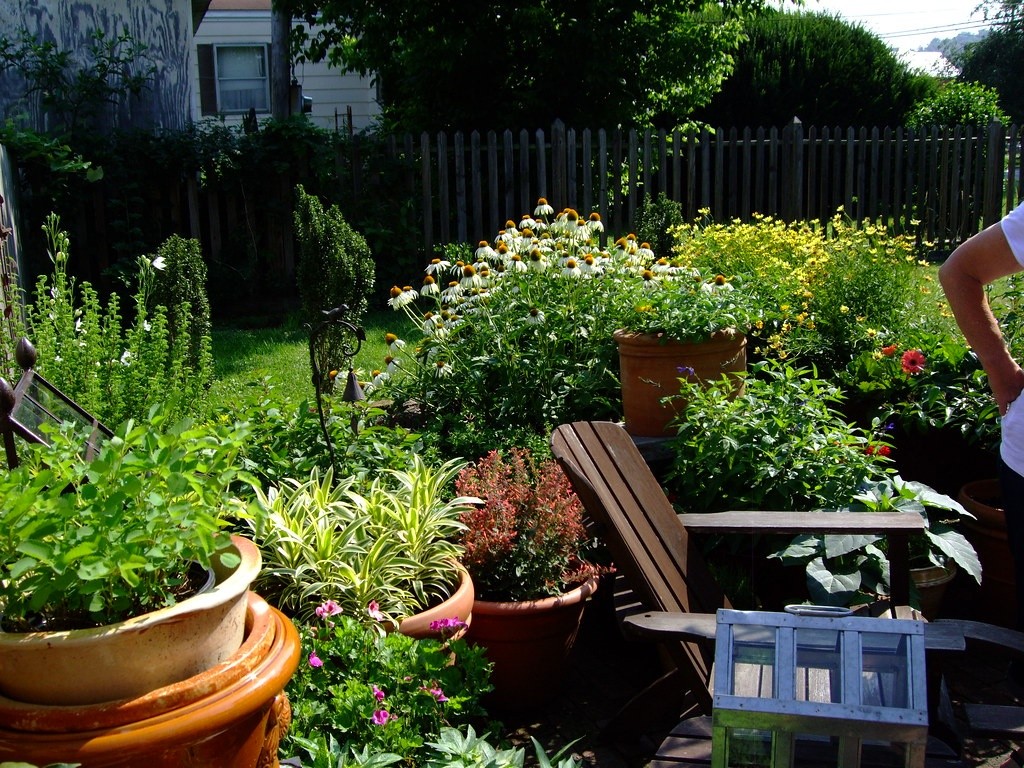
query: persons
[939,195,1024,705]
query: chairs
[549,421,966,731]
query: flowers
[608,276,764,346]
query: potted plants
[0,401,272,708]
[876,352,1023,616]
[450,446,618,701]
[848,476,984,623]
[230,452,484,671]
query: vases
[612,323,750,439]
